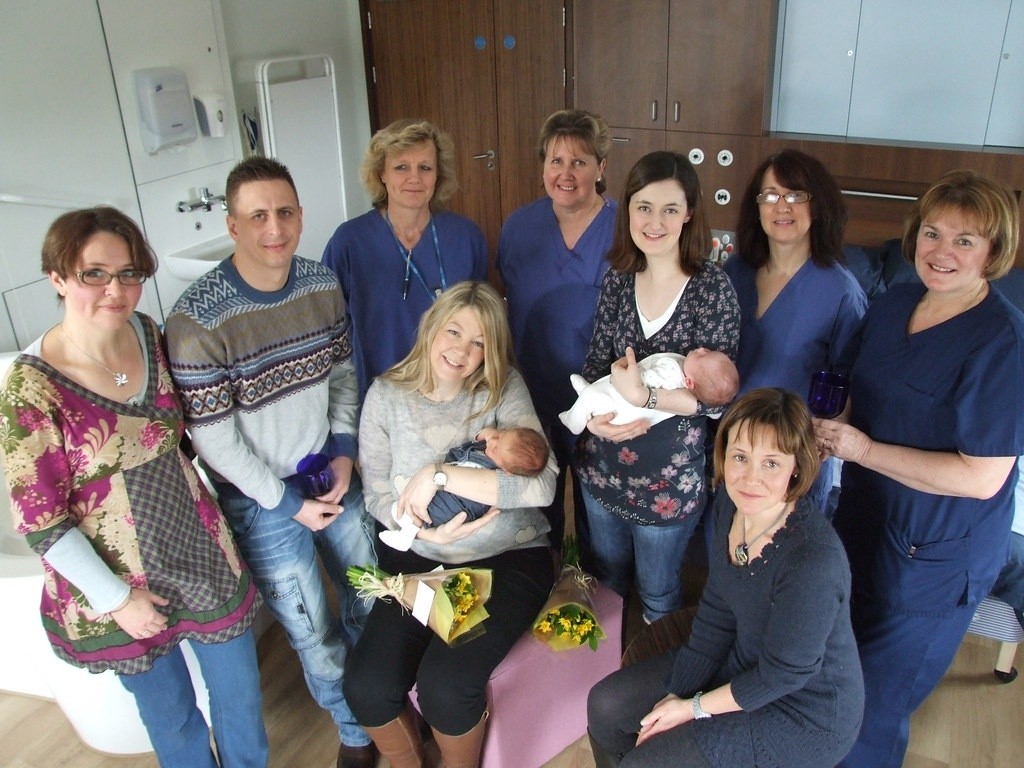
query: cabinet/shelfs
[501,1,566,230]
[759,136,1023,271]
[775,0,1010,154]
[984,0,1024,154]
[602,126,666,206]
[369,0,500,281]
[575,1,777,137]
[666,130,761,230]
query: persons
[558,346,740,435]
[497,110,619,569]
[570,152,741,638]
[704,149,867,576]
[318,119,488,413]
[810,171,1024,768]
[378,426,550,552]
[165,157,428,768]
[586,388,865,768]
[342,284,559,768]
[0,208,268,768]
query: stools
[402,564,624,767]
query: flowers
[533,533,606,650]
[347,561,489,644]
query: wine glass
[297,453,344,518]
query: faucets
[176,186,227,213]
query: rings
[822,440,826,447]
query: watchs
[692,691,712,720]
[433,463,447,491]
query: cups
[809,370,850,418]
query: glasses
[73,269,147,286]
[756,192,812,205]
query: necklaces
[736,499,791,565]
[60,324,127,385]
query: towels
[241,112,261,160]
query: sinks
[165,230,237,281]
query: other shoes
[337,742,377,768]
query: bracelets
[112,588,132,613]
[642,387,657,409]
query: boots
[428,701,489,768]
[361,695,432,768]
